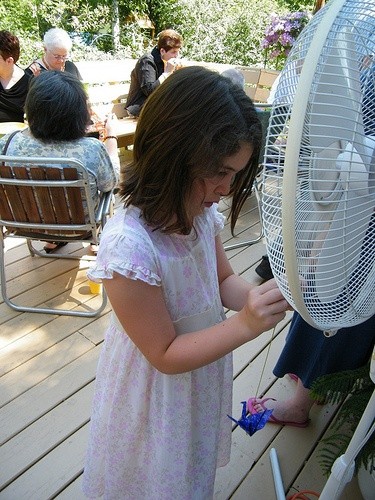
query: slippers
[248,396,311,428]
[289,372,327,406]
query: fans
[254,0,375,500]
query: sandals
[43,241,68,253]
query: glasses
[51,55,70,61]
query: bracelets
[103,136,119,141]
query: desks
[102,115,140,148]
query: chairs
[0,155,113,319]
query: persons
[0,29,34,124]
[247,68,375,429]
[76,64,292,500]
[25,28,92,125]
[0,70,121,253]
[124,29,182,116]
